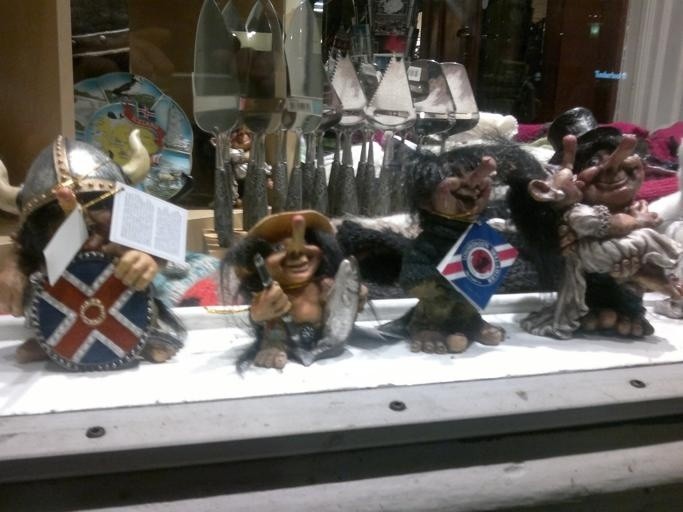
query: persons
[0,127,190,374]
[338,144,505,356]
[497,134,682,321]
[520,106,662,339]
[207,120,277,206]
[217,204,370,371]
